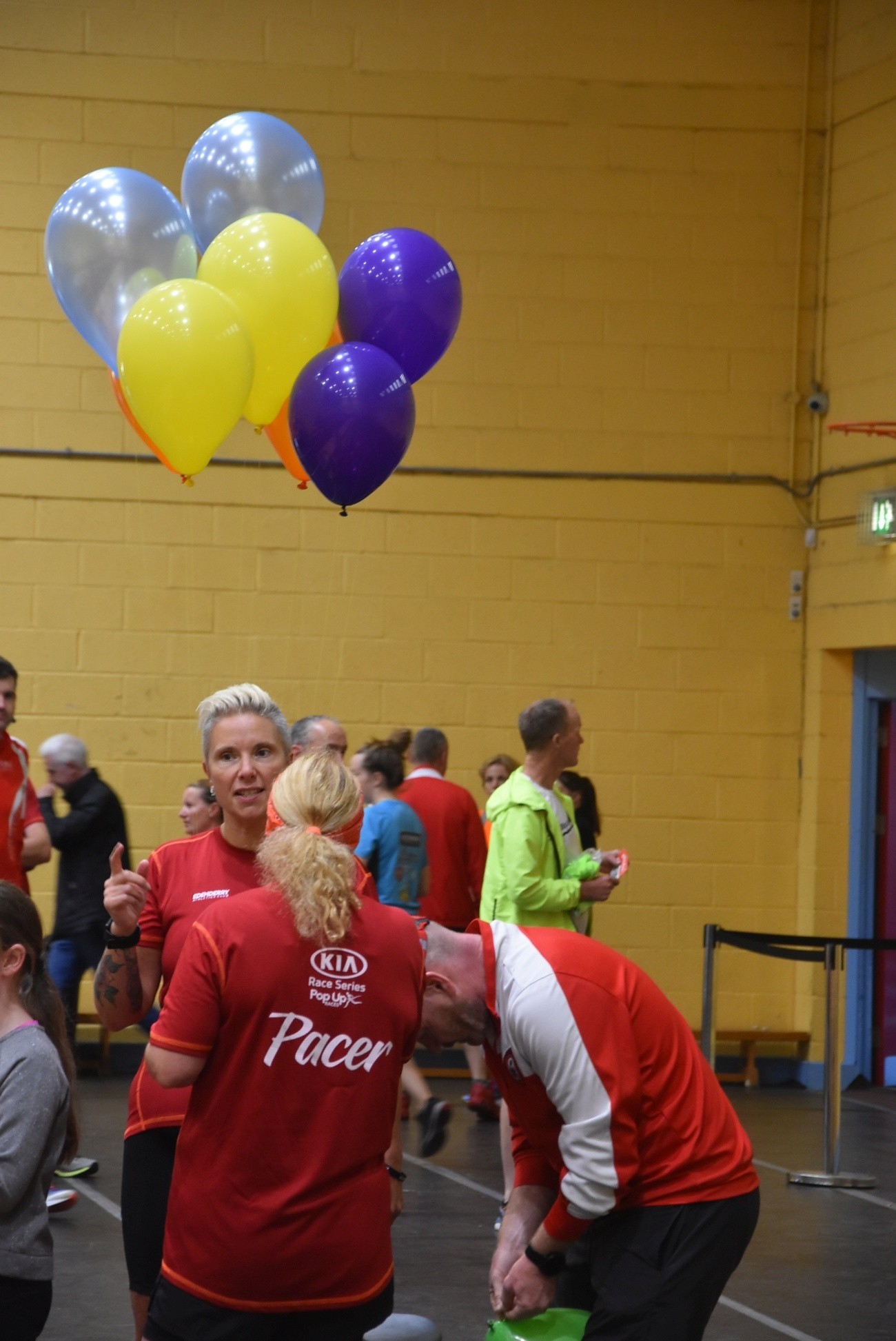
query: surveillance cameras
[807,392,827,414]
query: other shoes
[493,1201,508,1229]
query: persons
[0,878,80,1341]
[143,747,426,1341]
[94,682,379,1341]
[416,916,762,1341]
[0,655,602,1223]
[480,695,623,938]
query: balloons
[42,110,463,519]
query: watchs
[523,1240,567,1279]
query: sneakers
[54,1157,98,1177]
[415,1096,452,1157]
[400,1095,410,1121]
[46,1185,77,1212]
[467,1082,501,1120]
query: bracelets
[104,918,143,951]
[383,1162,408,1182]
